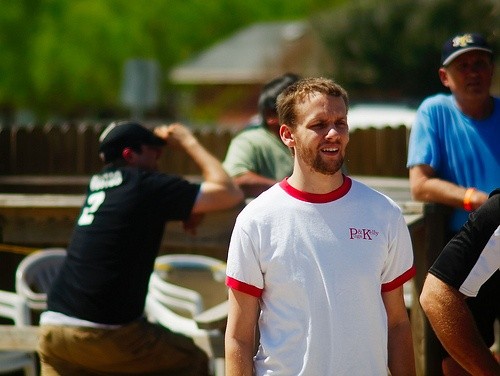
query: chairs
[0,246,229,376]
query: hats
[441,32,493,70]
[100,120,168,165]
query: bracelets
[463,188,475,211]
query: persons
[223,72,299,200]
[35,118,246,376]
[406,27,500,376]
[419,188,500,376]
[223,77,416,375]
